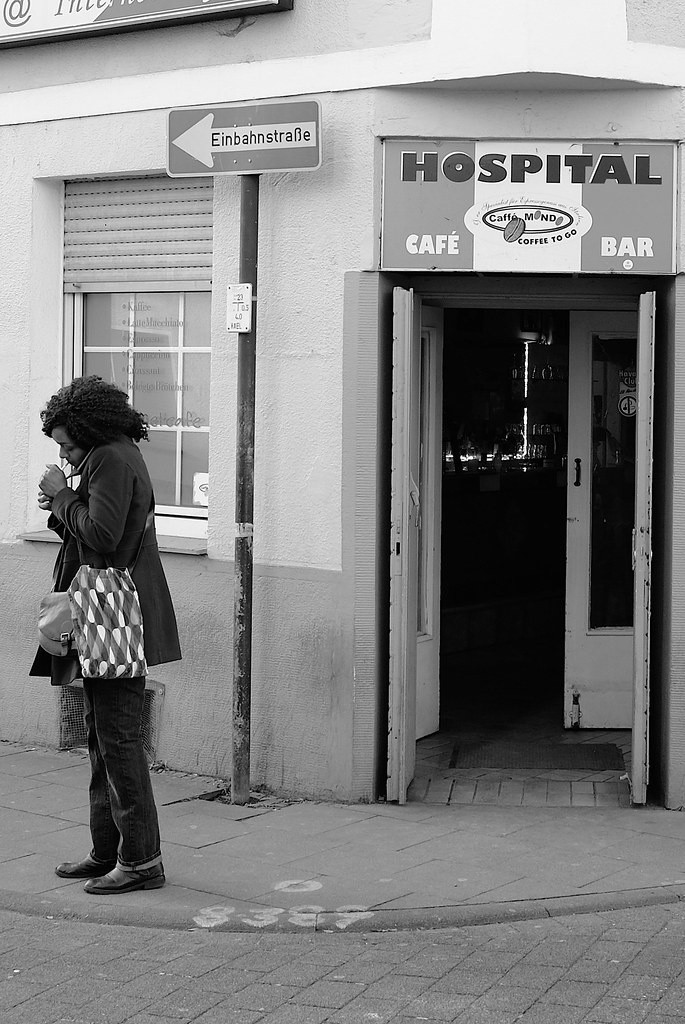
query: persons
[28,377,181,896]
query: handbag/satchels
[38,592,75,656]
[74,511,150,678]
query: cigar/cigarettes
[62,461,69,470]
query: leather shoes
[55,853,117,878]
[83,861,165,895]
